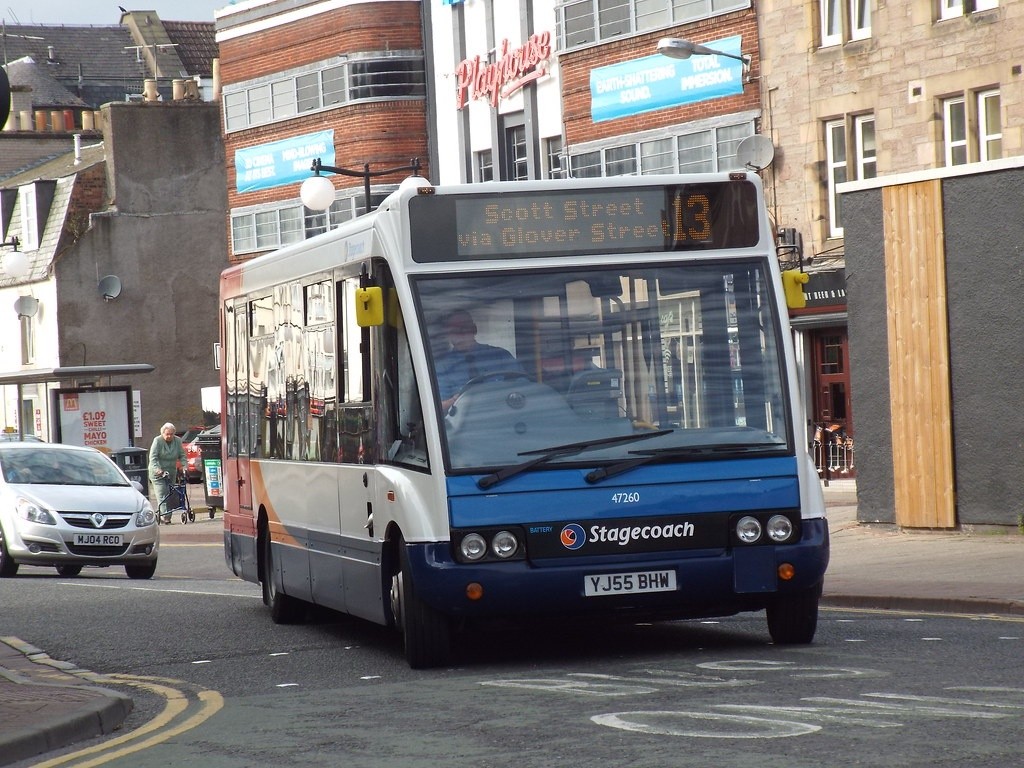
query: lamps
[656,37,752,73]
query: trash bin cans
[105,445,150,503]
[194,426,223,520]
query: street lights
[299,159,434,216]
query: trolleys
[155,471,197,524]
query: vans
[176,425,211,484]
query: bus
[216,165,830,669]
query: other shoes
[160,516,171,524]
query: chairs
[568,366,623,416]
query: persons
[432,310,526,412]
[149,422,187,524]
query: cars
[0,441,161,580]
[0,432,45,442]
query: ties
[465,357,482,384]
